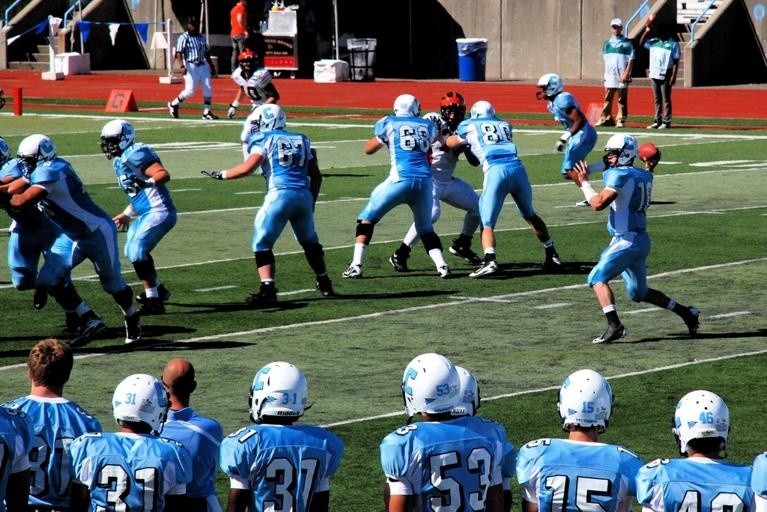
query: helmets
[400,352,461,418]
[250,103,287,135]
[671,387,731,459]
[14,134,57,179]
[111,373,173,437]
[440,90,466,117]
[97,117,136,160]
[450,365,482,417]
[0,136,11,167]
[393,93,420,118]
[556,369,615,435]
[470,100,496,119]
[536,72,564,100]
[602,133,638,170]
[248,360,310,425]
[237,48,258,72]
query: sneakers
[591,324,627,345]
[437,265,454,279]
[135,298,167,317]
[387,248,410,272]
[683,305,701,338]
[542,253,564,269]
[468,253,499,279]
[33,288,48,310]
[245,281,278,307]
[614,120,624,128]
[313,271,337,298]
[657,122,670,130]
[167,101,181,119]
[646,122,658,129]
[66,310,107,349]
[594,118,610,127]
[135,284,171,302]
[576,199,590,206]
[201,110,219,120]
[448,238,483,266]
[123,311,143,345]
[341,263,364,279]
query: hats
[610,18,622,27]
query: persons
[536,73,610,179]
[388,92,480,272]
[226,52,280,174]
[70,373,187,512]
[0,403,32,512]
[341,93,451,279]
[219,362,344,512]
[231,0,249,73]
[245,25,268,67]
[515,369,645,512]
[640,20,681,131]
[595,19,636,128]
[0,134,141,351]
[451,366,516,512]
[150,357,222,512]
[201,104,334,305]
[635,390,755,512]
[378,353,495,512]
[447,101,563,279]
[168,18,219,122]
[574,134,700,344]
[0,136,80,331]
[101,119,177,317]
[5,339,102,512]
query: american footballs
[635,141,662,163]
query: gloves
[554,130,571,152]
[130,174,156,189]
[226,104,238,119]
[200,169,228,180]
